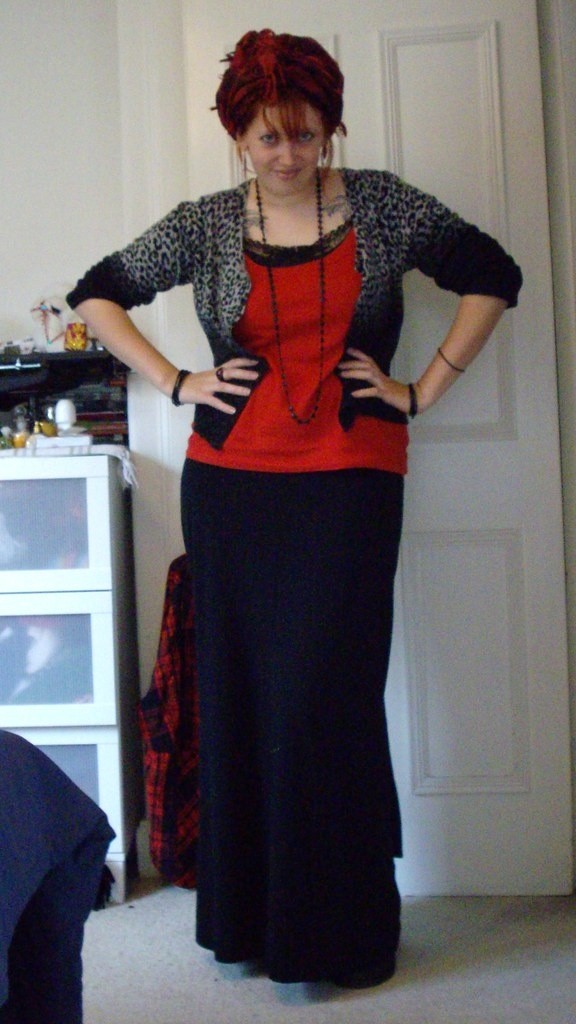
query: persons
[65,30,525,1002]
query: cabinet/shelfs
[0,452,143,907]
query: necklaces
[254,168,326,425]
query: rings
[216,367,224,382]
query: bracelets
[409,384,417,420]
[171,370,192,407]
[437,347,464,373]
